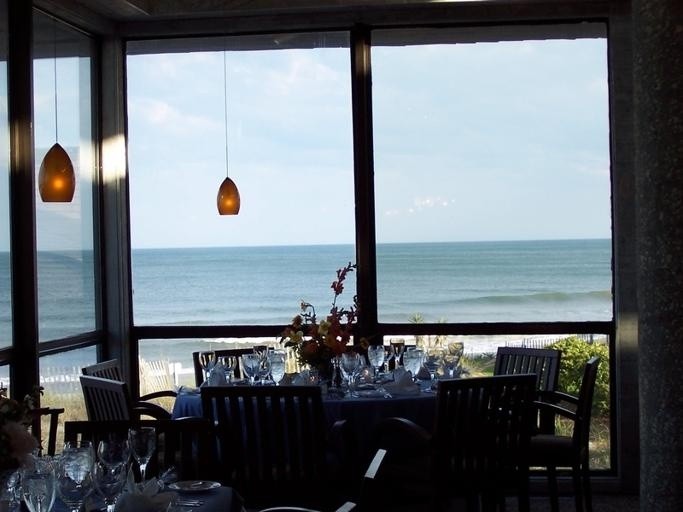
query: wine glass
[170,480,222,492]
[403,342,464,392]
[368,346,385,384]
[199,353,216,386]
[20,440,131,512]
[242,353,286,385]
[220,358,237,387]
[381,345,393,375]
[339,353,366,399]
[128,427,156,487]
[392,340,405,371]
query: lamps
[217,0,240,216]
[38,1,75,202]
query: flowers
[277,261,380,384]
